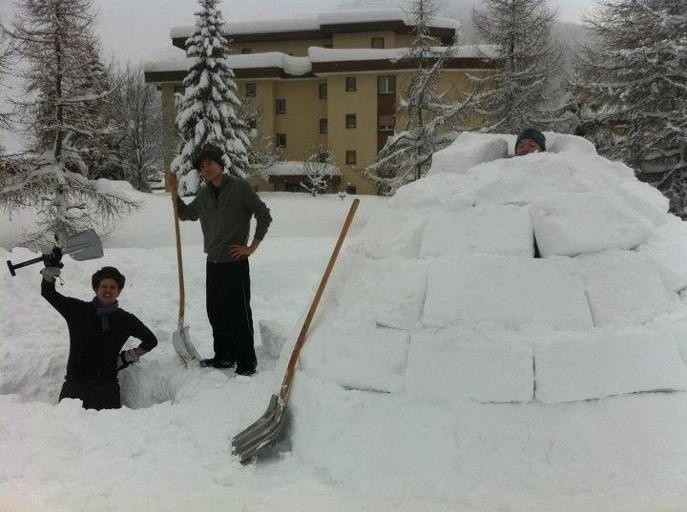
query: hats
[192,144,224,168]
[93,267,125,289]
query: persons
[514,128,547,156]
[40,246,158,413]
[165,150,273,377]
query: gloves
[116,348,139,370]
[40,248,64,282]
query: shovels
[231,198,360,464]
[170,172,202,368]
[7,228,104,277]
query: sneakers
[235,359,257,375]
[201,353,235,368]
